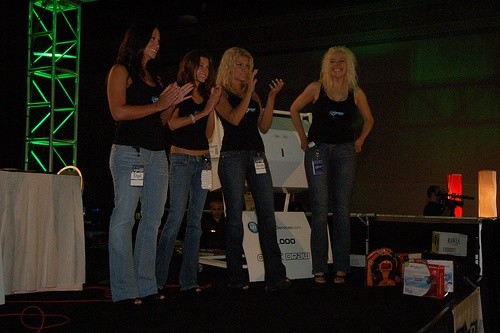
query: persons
[423,185,447,216]
[156,50,220,295]
[205,201,226,248]
[106,23,194,306]
[290,47,374,283]
[214,48,289,292]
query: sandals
[333,274,347,283]
[314,271,327,283]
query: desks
[0,169,85,305]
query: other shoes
[113,299,145,308]
[264,278,291,293]
[224,278,250,292]
[158,287,168,299]
[144,294,169,304]
[179,285,204,297]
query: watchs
[189,114,196,124]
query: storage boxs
[429,230,468,258]
[402,262,446,299]
